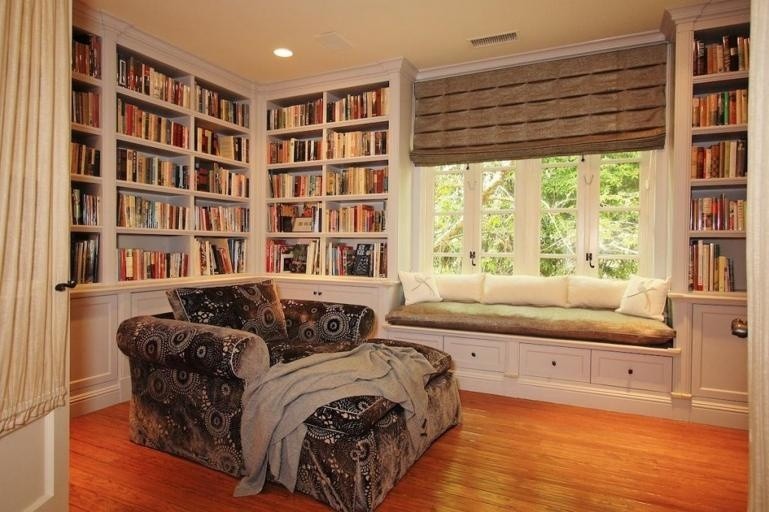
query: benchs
[385,301,680,421]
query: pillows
[399,270,670,322]
[165,278,295,364]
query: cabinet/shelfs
[670,3,750,429]
[71,20,110,389]
[108,32,259,376]
[261,78,396,335]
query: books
[266,87,389,279]
[70,38,101,285]
[195,84,251,276]
[116,55,191,282]
[687,34,749,293]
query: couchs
[108,297,462,511]
